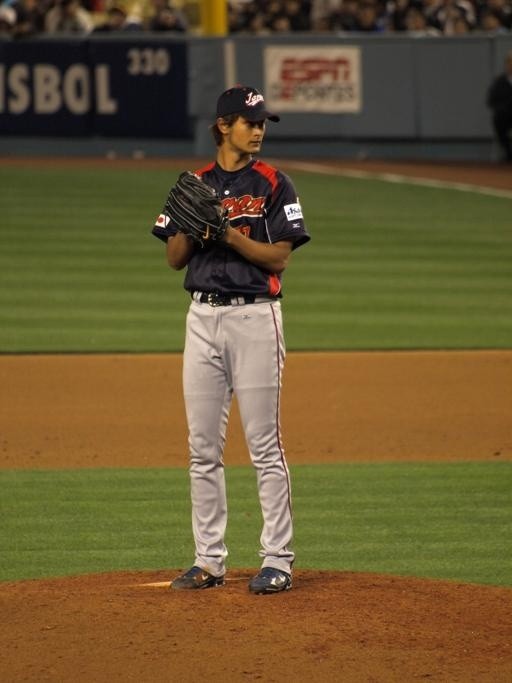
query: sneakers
[168,562,228,592]
[248,562,293,596]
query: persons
[485,54,512,163]
[151,85,310,591]
[1,1,511,41]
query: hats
[214,85,280,123]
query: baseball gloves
[162,171,229,248]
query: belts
[191,289,279,306]
